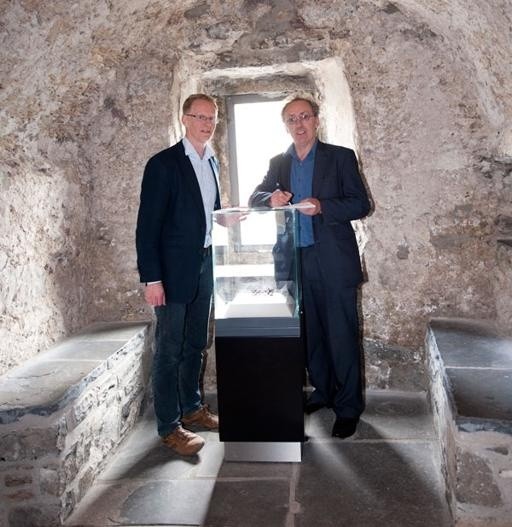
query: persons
[247,95,376,439]
[133,93,252,456]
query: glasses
[185,113,219,124]
[287,112,316,124]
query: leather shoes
[332,416,359,439]
[305,397,323,414]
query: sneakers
[181,404,219,432]
[160,426,204,456]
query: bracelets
[146,280,162,286]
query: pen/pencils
[276,182,291,205]
